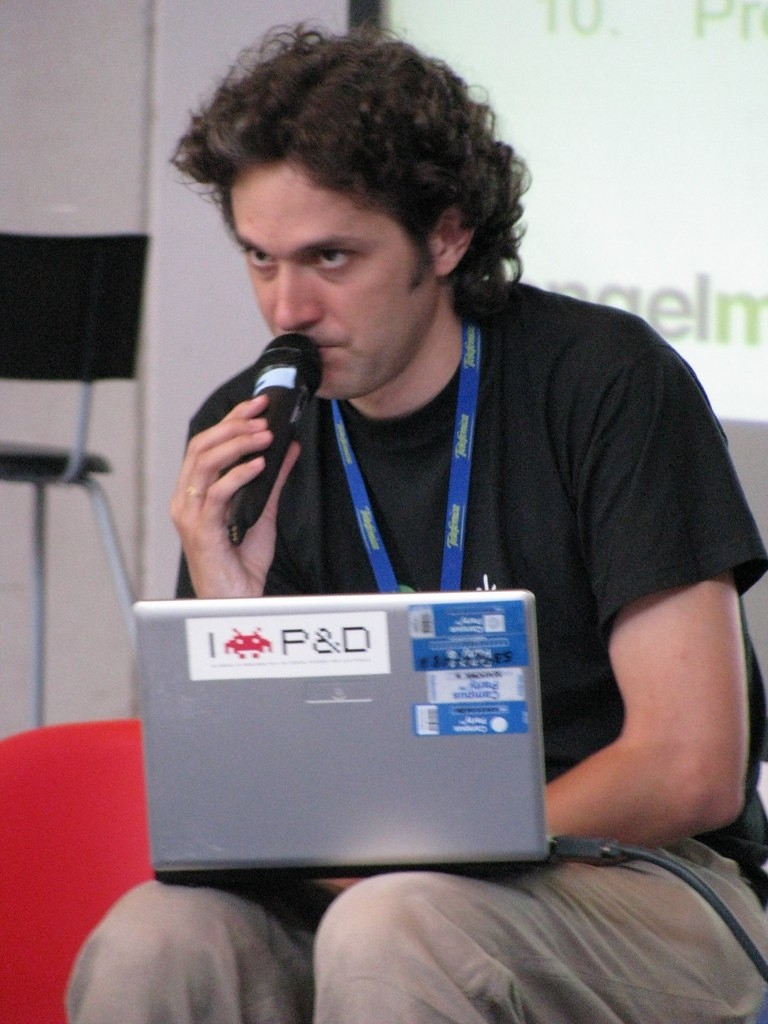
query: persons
[65,23,768,1024]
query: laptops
[130,590,559,885]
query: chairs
[1,233,151,728]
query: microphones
[228,332,324,545]
